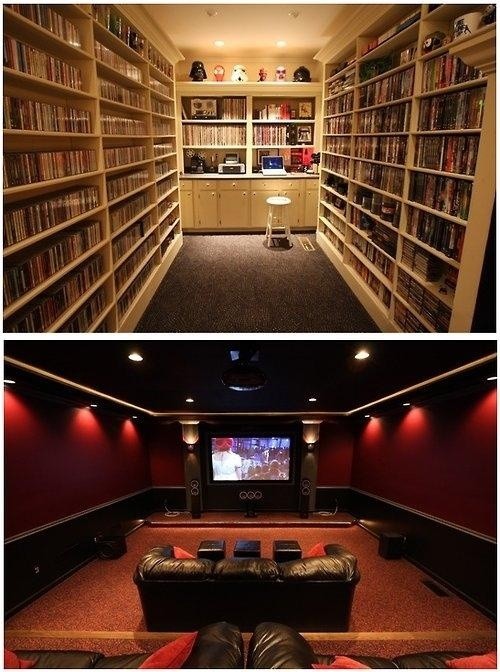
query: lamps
[354,347,372,363]
[127,348,143,363]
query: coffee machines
[190,154,205,174]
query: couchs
[133,535,362,635]
[250,618,496,669]
[5,613,245,668]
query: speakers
[190,478,202,519]
[239,491,264,501]
[300,478,311,519]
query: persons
[211,438,242,480]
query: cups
[99,6,144,57]
[453,12,483,40]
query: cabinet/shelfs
[173,73,323,173]
[3,3,190,333]
[178,172,322,238]
[307,3,496,330]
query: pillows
[443,646,496,669]
[4,645,41,669]
[172,546,195,560]
[310,650,379,669]
[301,543,328,559]
[138,625,202,668]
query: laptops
[261,155,288,176]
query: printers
[218,161,246,174]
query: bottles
[292,66,310,82]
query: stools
[261,194,298,250]
[271,538,305,563]
[234,537,265,558]
[377,529,407,562]
[196,538,226,563]
